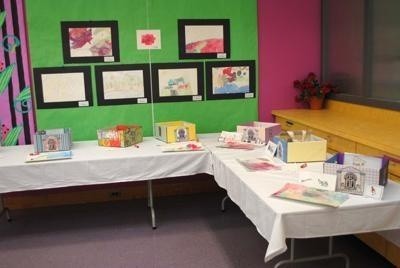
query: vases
[309,96,324,108]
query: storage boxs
[33,128,72,153]
[154,121,195,144]
[322,152,389,187]
[97,125,142,148]
[236,122,282,145]
[274,135,327,163]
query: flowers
[293,72,338,101]
[329,72,351,92]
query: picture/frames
[94,63,152,106]
[136,30,161,50]
[205,60,256,100]
[33,66,93,110]
[177,18,230,59]
[60,20,120,64]
[151,62,204,103]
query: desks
[0,137,213,230]
[271,100,399,183]
[197,132,400,268]
